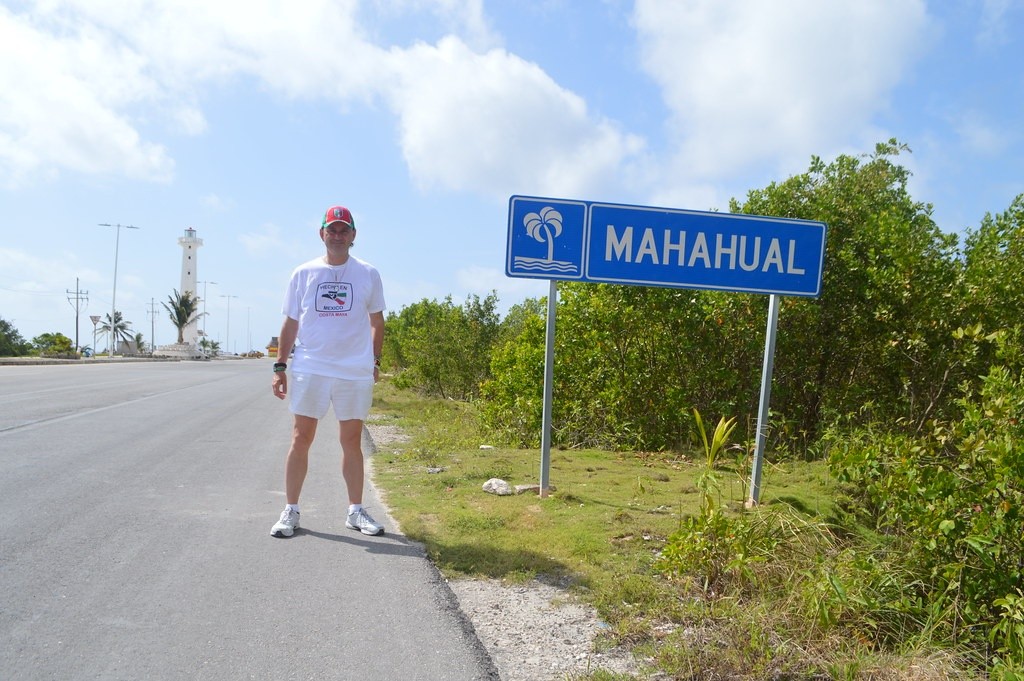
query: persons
[269,206,387,537]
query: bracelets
[372,353,381,369]
[274,362,287,368]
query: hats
[321,205,355,230]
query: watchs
[274,367,286,372]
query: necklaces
[325,253,349,292]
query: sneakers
[346,507,385,536]
[270,507,301,538]
